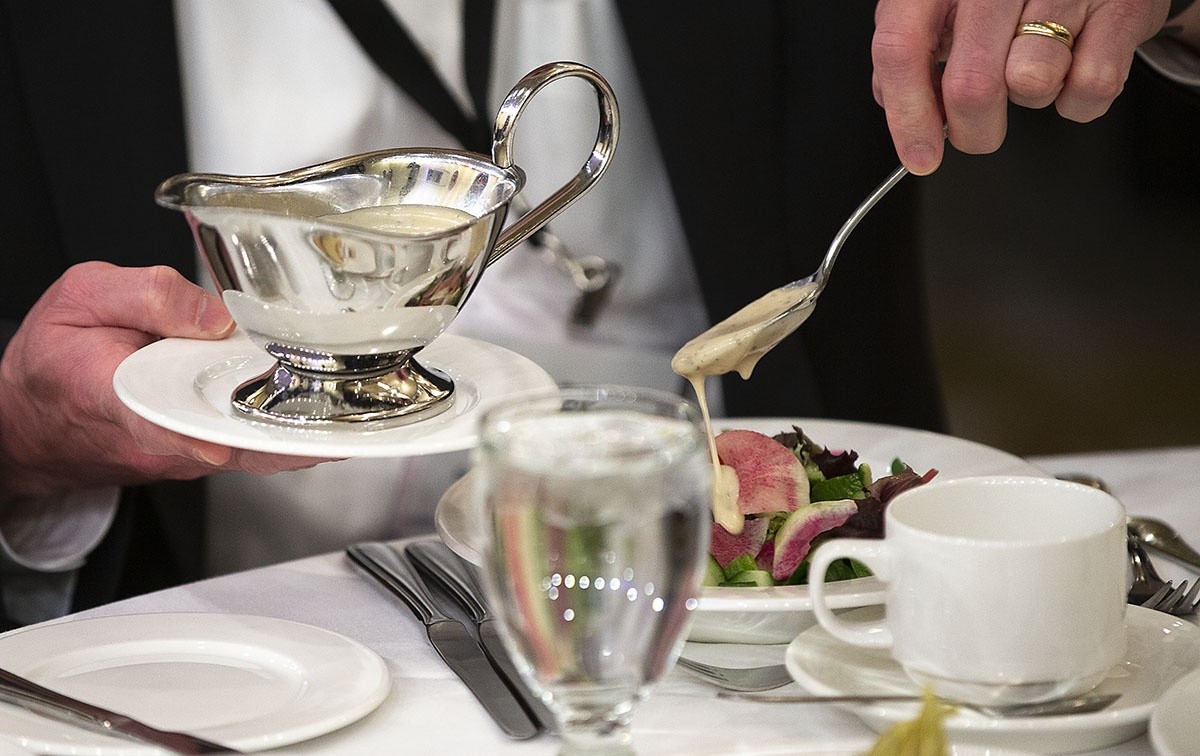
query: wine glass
[471,386,714,756]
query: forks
[673,656,795,692]
[1056,473,1200,616]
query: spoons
[715,689,1123,718]
[672,165,909,377]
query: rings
[1015,21,1075,51]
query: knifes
[343,540,560,742]
[0,668,245,756]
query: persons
[0,0,1200,635]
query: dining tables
[0,448,1200,754]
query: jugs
[152,62,620,433]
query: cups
[808,476,1130,708]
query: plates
[434,417,1058,644]
[112,323,564,458]
[0,611,393,756]
[783,601,1199,755]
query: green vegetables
[706,425,924,586]
